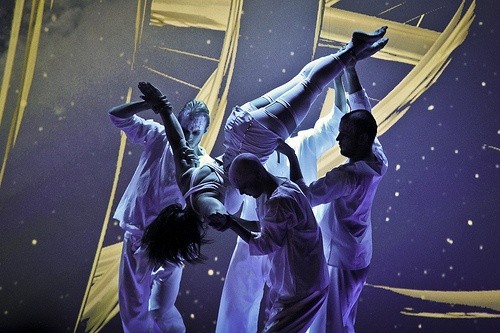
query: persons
[215,67,348,333]
[137,24,391,247]
[276,60,387,333]
[219,151,331,333]
[108,95,219,333]
